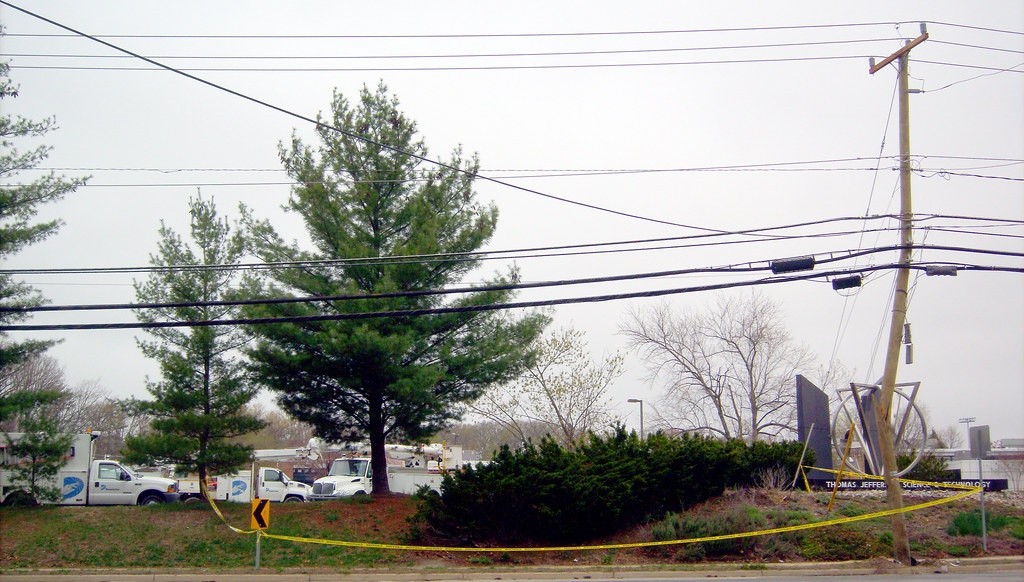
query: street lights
[958,416,976,449]
[627,398,644,442]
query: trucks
[175,457,312,504]
[0,426,182,510]
[305,454,456,501]
